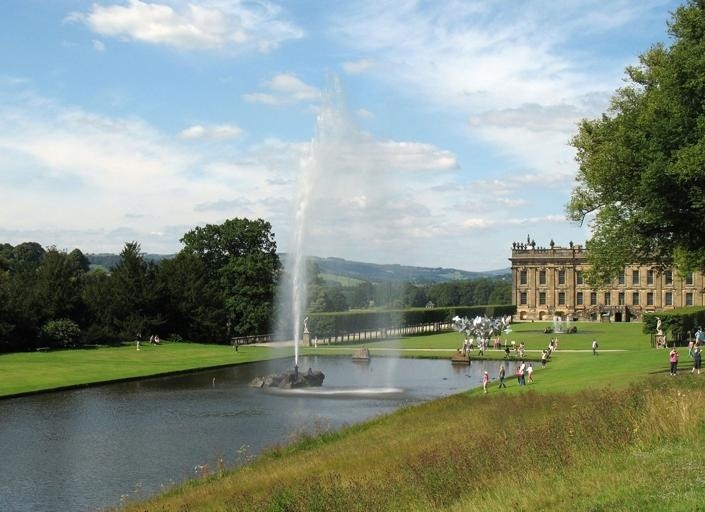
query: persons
[314,336,318,347]
[592,338,599,356]
[695,328,702,346]
[150,334,156,344]
[233,340,239,352]
[155,335,160,343]
[457,336,559,394]
[688,340,696,357]
[670,348,679,376]
[691,347,703,375]
[137,332,142,350]
